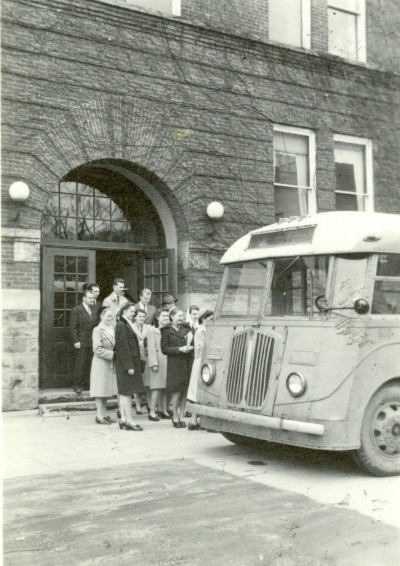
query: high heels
[172,418,180,428]
[119,419,142,430]
[148,415,159,421]
[117,410,121,418]
[104,416,116,424]
[136,406,142,416]
[96,415,111,424]
[180,420,187,428]
[157,409,173,418]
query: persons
[160,308,194,428]
[185,305,199,334]
[102,278,130,314]
[90,306,118,425]
[114,302,145,430]
[71,290,97,393]
[147,308,172,421]
[134,289,156,324]
[133,309,147,373]
[161,294,178,310]
[186,311,214,430]
[88,284,102,314]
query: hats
[97,307,108,319]
[162,294,178,303]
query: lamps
[8,181,30,200]
[206,200,225,219]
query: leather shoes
[189,423,204,430]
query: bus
[185,210,400,476]
